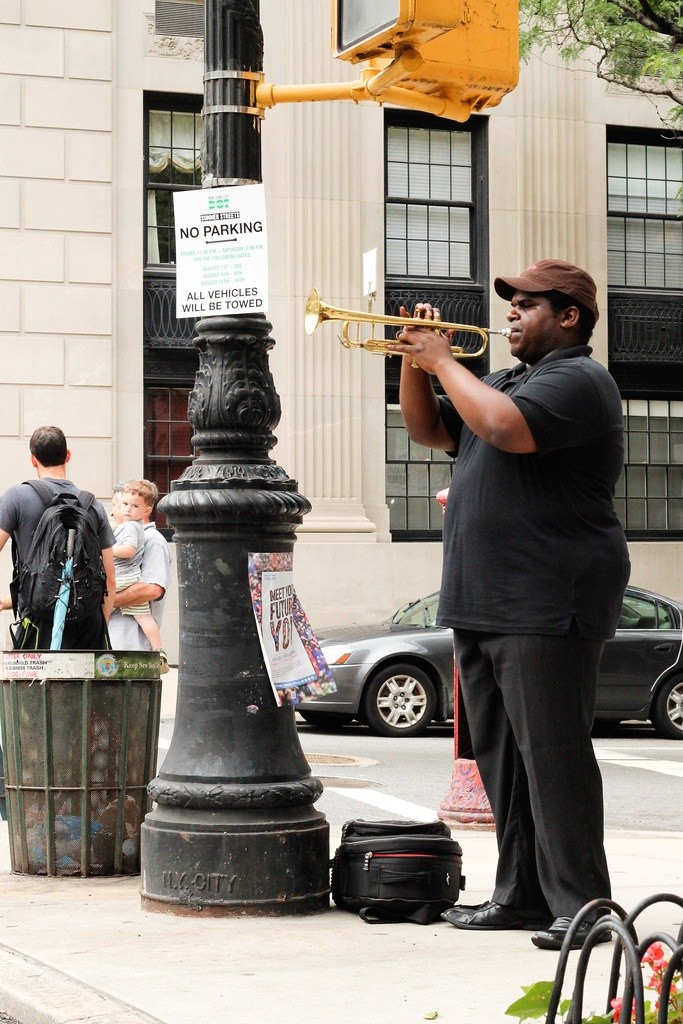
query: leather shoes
[440,901,552,930]
[531,917,612,950]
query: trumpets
[301,289,510,369]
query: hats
[494,259,600,324]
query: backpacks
[331,819,466,924]
[9,480,108,625]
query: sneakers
[155,648,169,674]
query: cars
[294,583,683,740]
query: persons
[1,597,14,613]
[103,477,171,675]
[0,425,117,650]
[390,259,635,950]
